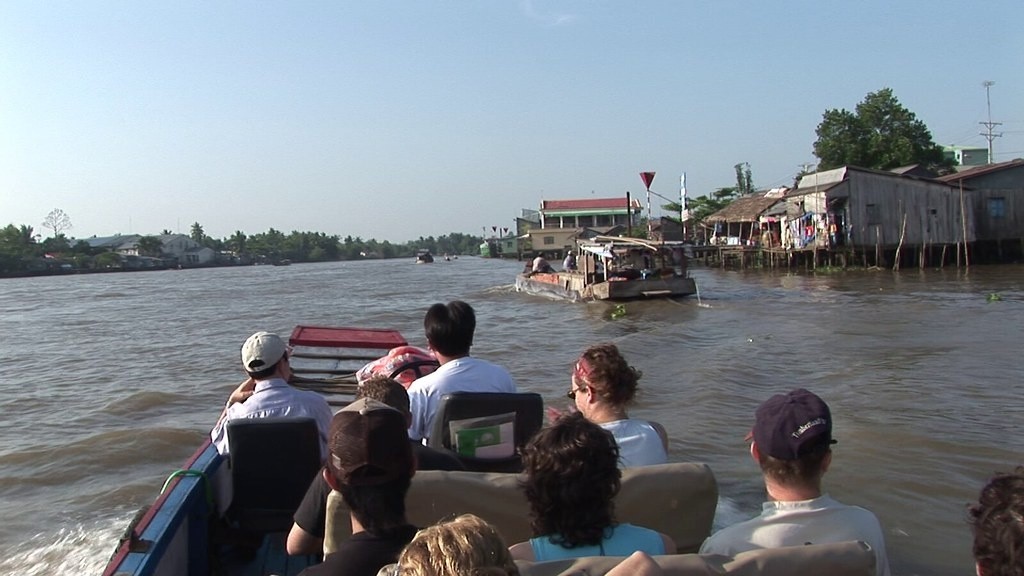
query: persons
[571,344,669,471]
[698,389,889,576]
[210,332,334,472]
[377,513,521,576]
[503,412,678,560]
[404,300,516,446]
[563,251,576,271]
[531,252,551,273]
[295,396,423,576]
[967,467,1024,576]
[286,376,462,555]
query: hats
[743,388,838,459]
[328,396,410,472]
[241,332,294,373]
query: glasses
[567,385,596,399]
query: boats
[416,248,435,264]
[101,324,878,576]
[515,235,697,301]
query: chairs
[223,415,322,565]
[430,391,543,454]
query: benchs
[322,461,878,576]
